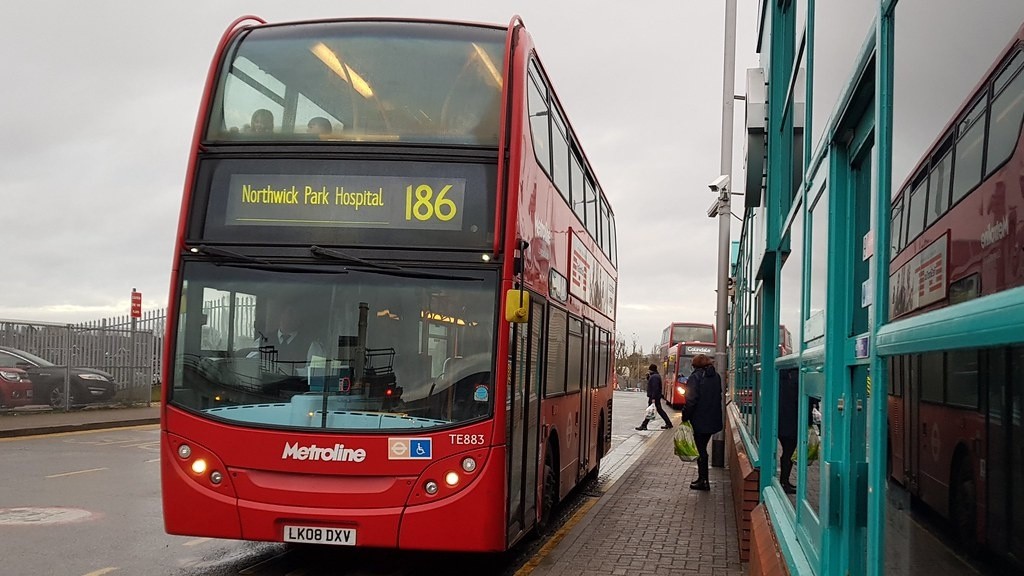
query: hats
[692,353,711,368]
[649,364,658,371]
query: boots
[690,455,710,491]
[779,457,796,494]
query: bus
[659,322,717,410]
[160,14,617,552]
[886,23,1024,571]
[737,324,793,412]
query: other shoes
[635,427,647,430]
[661,424,673,429]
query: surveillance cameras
[708,175,730,192]
[706,197,720,218]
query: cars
[0,347,117,411]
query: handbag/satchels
[812,408,821,424]
[673,420,699,462]
[790,426,819,467]
[644,403,657,419]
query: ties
[280,334,291,345]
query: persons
[682,353,723,490]
[251,108,274,134]
[244,298,327,378]
[306,117,332,135]
[778,367,822,493]
[635,364,674,430]
